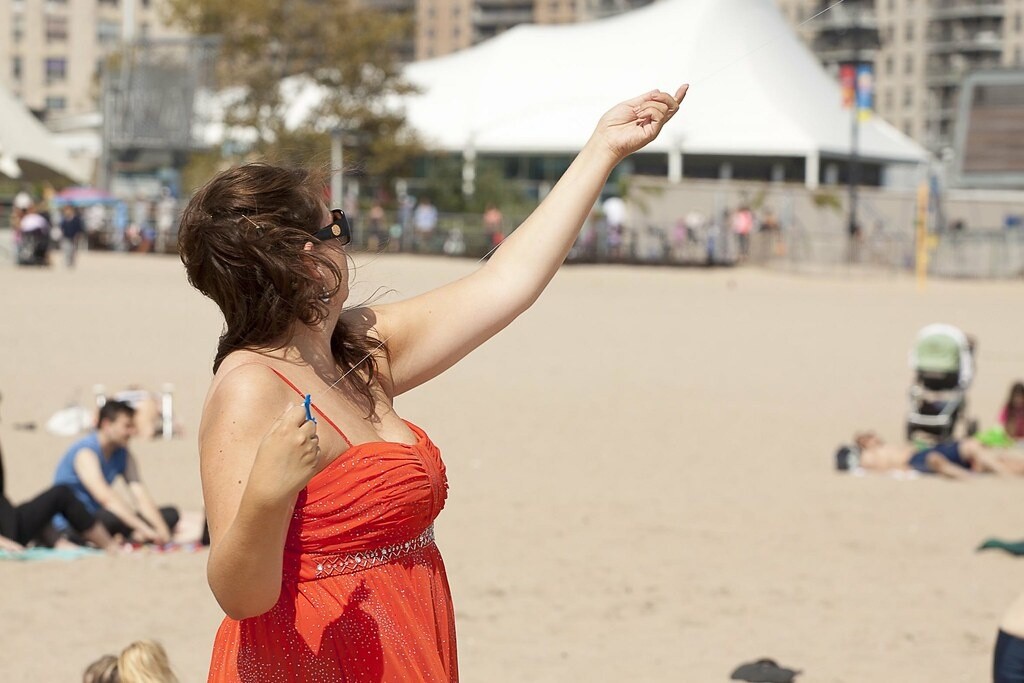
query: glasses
[307,208,350,245]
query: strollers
[906,322,977,446]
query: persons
[82,638,176,683]
[0,447,123,548]
[856,432,1024,478]
[9,184,758,267]
[176,82,691,683]
[998,381,1024,438]
[52,397,180,546]
